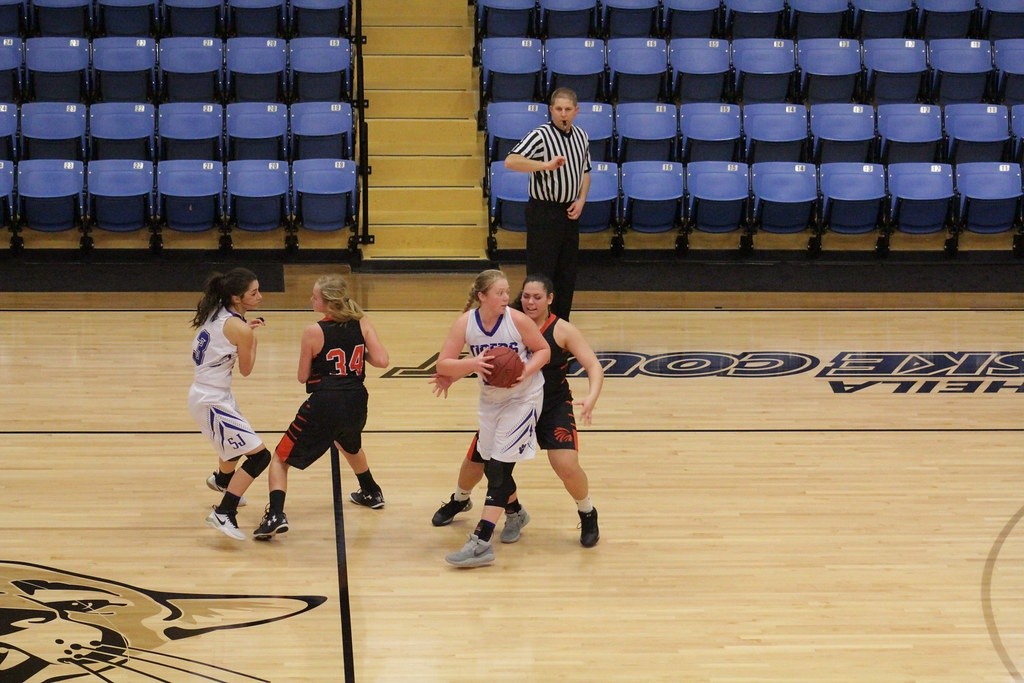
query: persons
[254,274,389,542]
[436,269,551,567]
[428,272,604,547]
[504,88,593,322]
[187,268,271,541]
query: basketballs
[484,347,523,388]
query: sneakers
[500,504,530,543]
[206,504,246,541]
[254,502,289,540]
[576,506,599,547]
[350,486,385,508]
[206,470,246,505]
[432,492,472,526]
[445,532,494,566]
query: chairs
[1,0,366,269]
[465,0,1024,256]
